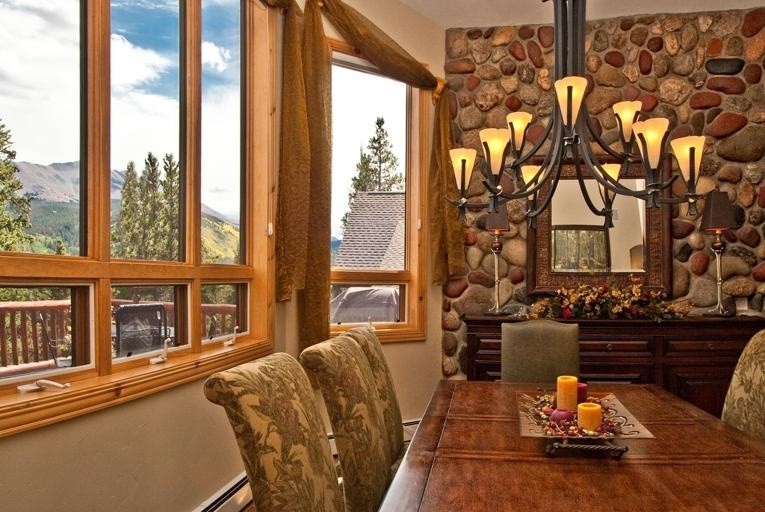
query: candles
[517,376,656,457]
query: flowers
[525,282,688,325]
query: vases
[525,282,688,325]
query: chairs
[721,316,764,435]
[38,302,168,369]
[502,320,579,384]
[201,350,342,512]
[299,326,410,510]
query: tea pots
[526,153,673,298]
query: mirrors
[526,153,673,298]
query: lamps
[38,302,168,369]
[483,194,511,315]
[698,190,738,318]
[443,0,713,219]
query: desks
[380,380,764,510]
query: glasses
[698,190,738,318]
[443,0,713,219]
[483,194,511,315]
